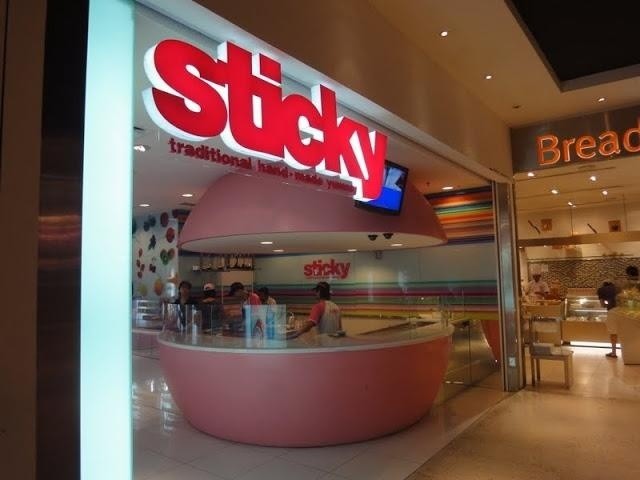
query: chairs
[526,318,580,391]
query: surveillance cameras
[384,233,392,239]
[368,234,378,240]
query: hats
[229,283,242,297]
[204,282,215,292]
[317,282,328,289]
[533,266,540,275]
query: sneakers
[607,353,616,356]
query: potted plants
[608,286,640,366]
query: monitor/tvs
[354,159,410,216]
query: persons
[524,271,551,299]
[276,281,342,339]
[197,283,226,336]
[171,281,198,332]
[229,280,265,332]
[621,265,640,293]
[597,279,640,366]
[257,285,277,324]
